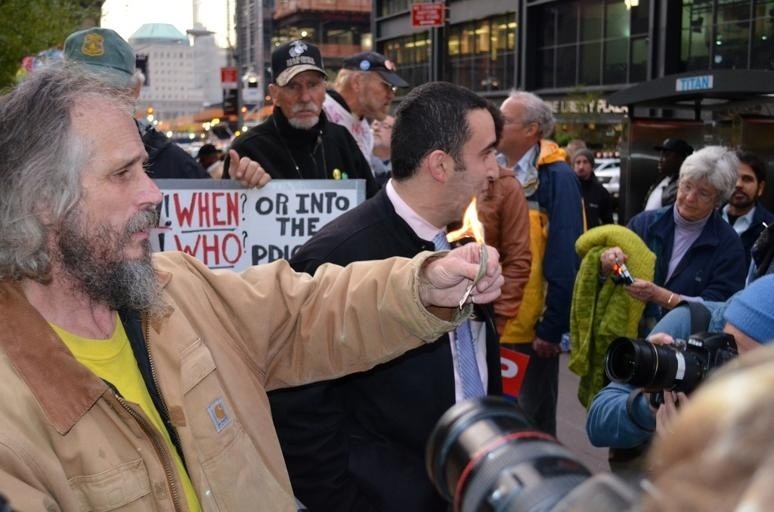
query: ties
[433,232,485,398]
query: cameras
[604,331,738,408]
[426,395,643,512]
[610,257,634,285]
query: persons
[322,50,410,190]
[0,60,508,511]
[446,90,618,438]
[63,28,272,189]
[266,83,503,511]
[598,133,773,511]
[195,143,227,180]
[221,40,379,201]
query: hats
[64,27,136,83]
[724,273,774,345]
[344,52,410,88]
[271,39,328,87]
